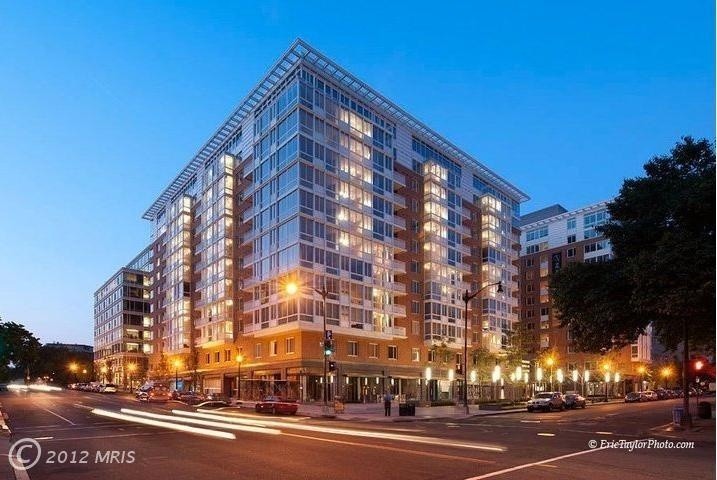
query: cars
[134,384,230,405]
[562,393,587,409]
[624,386,706,404]
[67,380,117,393]
[254,395,299,416]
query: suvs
[525,390,564,413]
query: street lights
[69,361,80,382]
[279,276,328,417]
[235,354,245,399]
[172,358,183,390]
[543,355,554,393]
[662,368,670,390]
[636,364,644,395]
[599,362,611,403]
[461,280,505,417]
[100,367,107,384]
[127,361,137,395]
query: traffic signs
[325,329,333,339]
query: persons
[382,391,392,416]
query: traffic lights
[324,340,332,357]
[329,361,336,372]
[695,359,706,374]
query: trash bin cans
[399,403,408,416]
[672,408,683,425]
[698,402,711,419]
[407,403,415,416]
